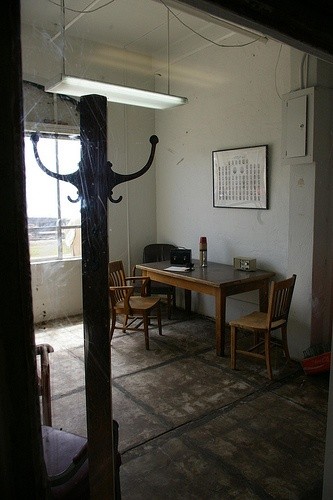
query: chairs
[108,260,162,351]
[36,344,122,500]
[142,244,177,320]
[229,274,296,381]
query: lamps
[45,0,189,111]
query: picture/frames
[211,144,269,210]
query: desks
[135,259,276,357]
